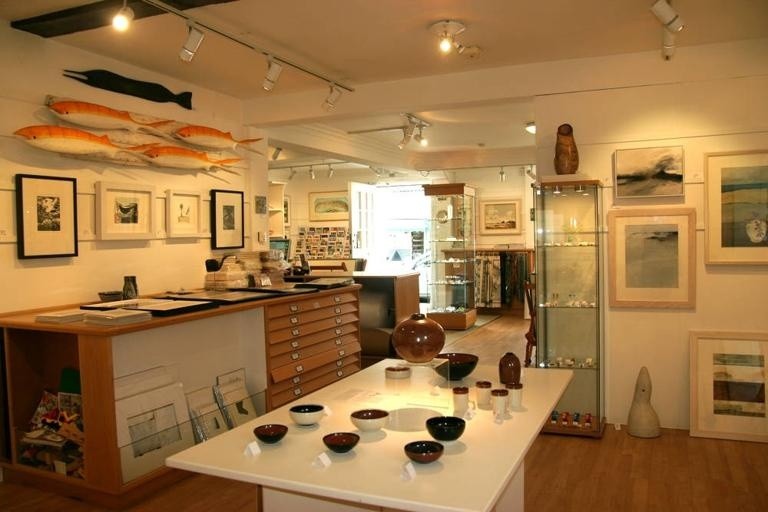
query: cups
[450,380,524,417]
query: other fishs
[143,146,243,175]
[175,126,264,155]
[13,125,162,168]
[48,101,176,141]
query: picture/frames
[92,179,159,242]
[613,144,686,199]
[702,147,768,267]
[12,172,79,259]
[209,188,244,250]
[681,329,768,445]
[606,204,696,312]
[163,188,202,239]
[117,379,197,486]
[477,199,521,235]
[306,189,350,222]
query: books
[295,226,352,258]
[35,308,154,330]
[205,250,263,289]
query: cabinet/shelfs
[532,180,606,439]
[423,183,478,330]
[1,278,362,512]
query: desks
[160,352,582,512]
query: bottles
[123,274,138,297]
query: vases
[392,311,446,364]
[123,276,140,310]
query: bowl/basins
[350,411,392,431]
[425,415,464,440]
[98,291,123,302]
[288,405,326,426]
[400,441,445,464]
[251,423,288,442]
[435,350,479,380]
[322,434,358,453]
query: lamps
[110,0,360,112]
[268,162,350,180]
[526,121,536,136]
[650,1,690,63]
[398,110,430,153]
[427,18,483,62]
